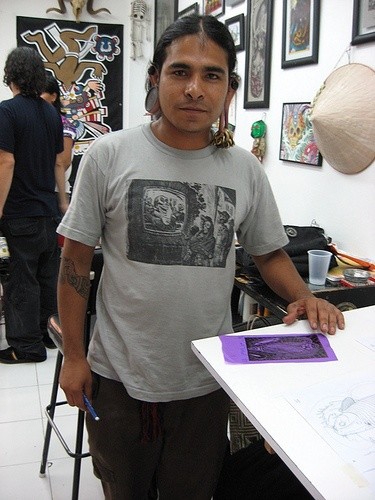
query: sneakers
[0,345,47,363]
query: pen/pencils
[81,392,100,422]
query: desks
[190,305,375,500]
[231,266,375,333]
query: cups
[308,249,332,285]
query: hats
[309,63,375,177]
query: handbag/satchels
[237,225,338,275]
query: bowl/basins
[344,268,369,283]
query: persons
[0,48,64,364]
[40,75,70,210]
[57,14,344,500]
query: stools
[39,317,91,500]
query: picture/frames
[153,0,375,167]
